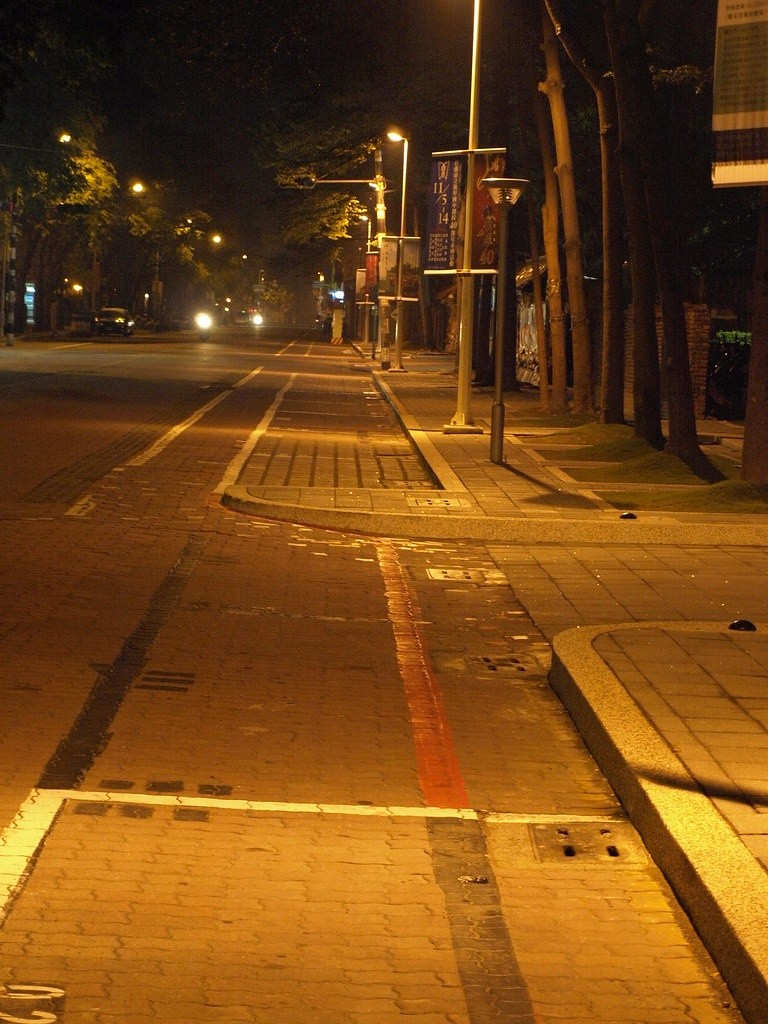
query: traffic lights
[280,174,317,188]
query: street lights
[387,132,409,372]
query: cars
[93,307,135,338]
